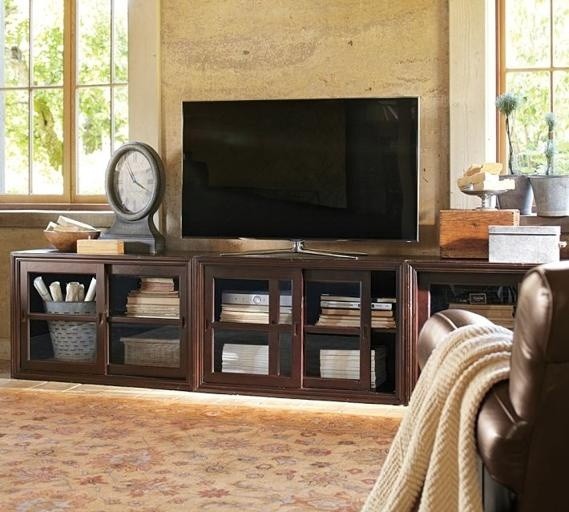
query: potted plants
[494,92,567,214]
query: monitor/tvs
[181,96,420,259]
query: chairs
[417,263,568,511]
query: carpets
[0,386,402,512]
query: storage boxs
[438,210,562,264]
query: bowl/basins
[42,230,101,252]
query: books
[448,302,516,330]
[218,291,399,390]
[122,276,182,321]
[45,216,97,231]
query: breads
[457,161,516,191]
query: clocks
[103,141,165,222]
